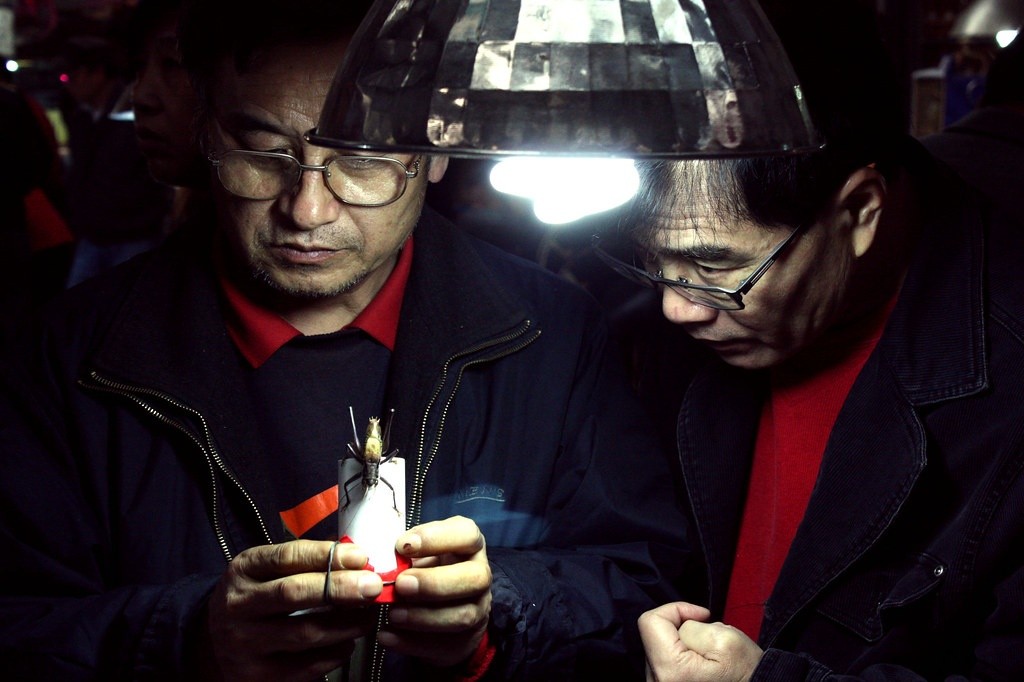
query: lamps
[303,1,827,227]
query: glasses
[590,162,879,311]
[201,104,421,207]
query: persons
[1,0,688,682]
[622,1,1024,682]
